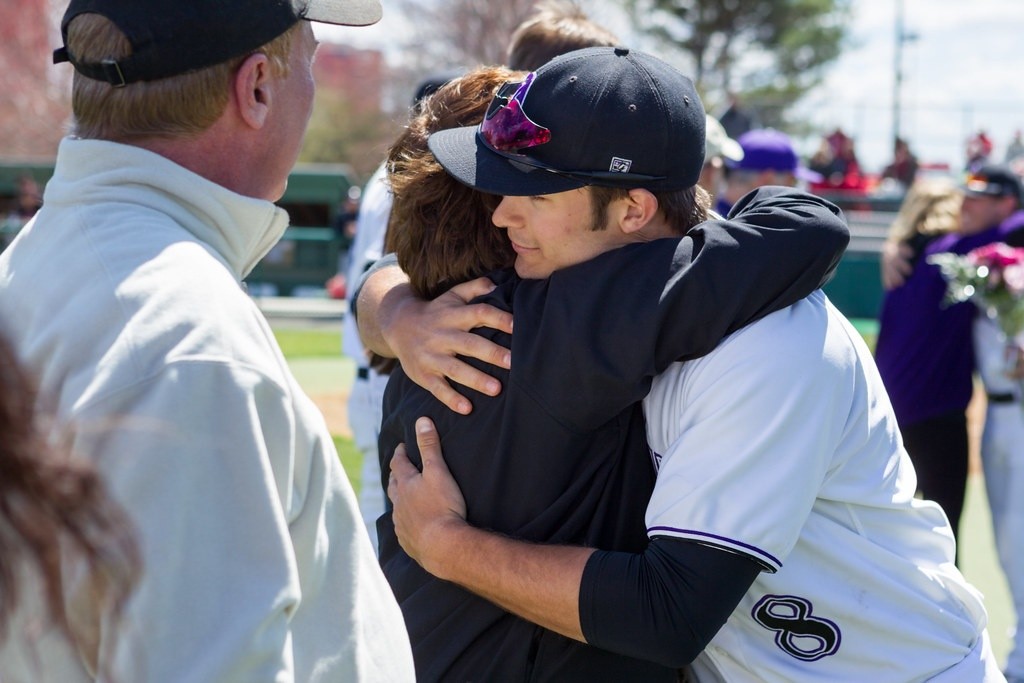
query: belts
[988,393,1019,406]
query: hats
[723,128,823,184]
[426,45,706,197]
[53,1,381,87]
[957,165,1020,197]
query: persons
[874,161,1024,683]
[342,1,1009,683]
[0,0,416,682]
